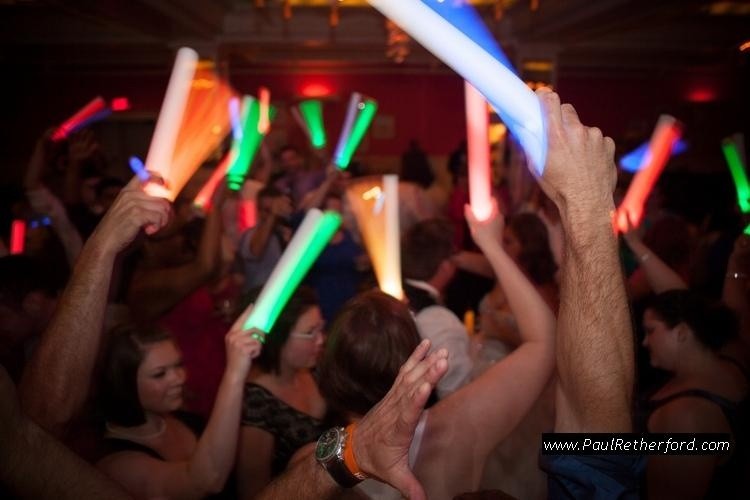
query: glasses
[289,320,325,339]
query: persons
[1,76,750,499]
